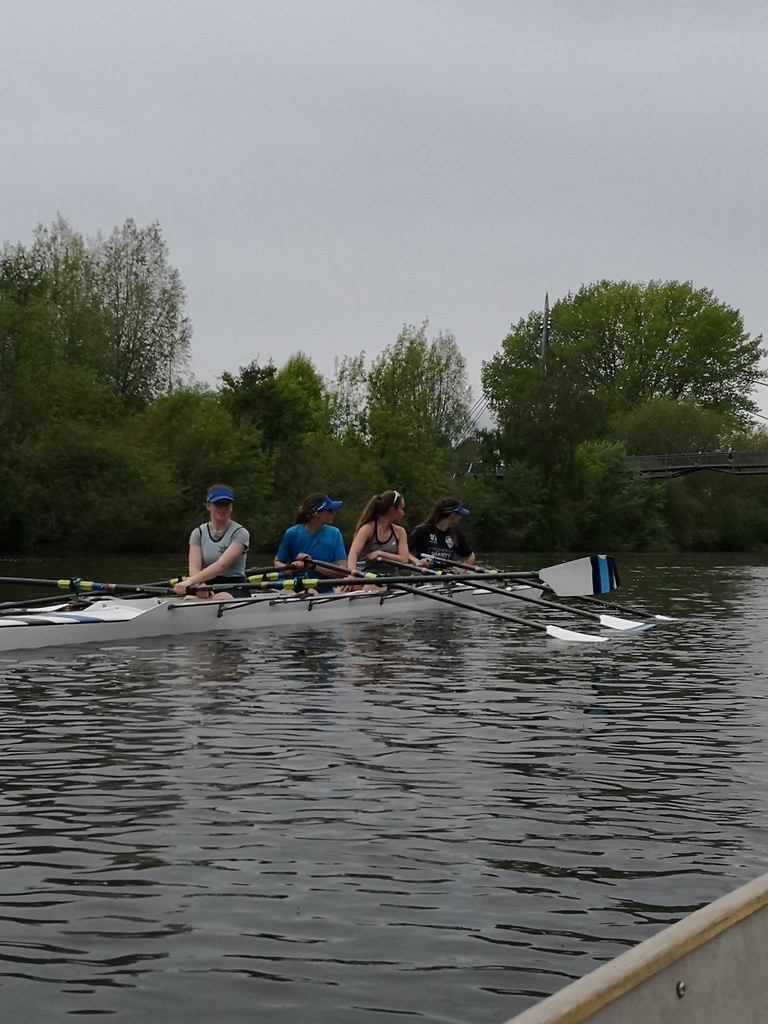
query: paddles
[1,575,188,609]
[421,554,680,622]
[377,557,656,634]
[304,556,611,644]
[186,552,621,598]
[0,577,176,595]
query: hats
[443,502,470,515]
[207,487,235,502]
[309,494,342,516]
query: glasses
[393,490,401,503]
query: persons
[274,494,348,595]
[408,498,475,574]
[173,485,250,600]
[336,491,410,594]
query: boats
[0,570,547,652]
